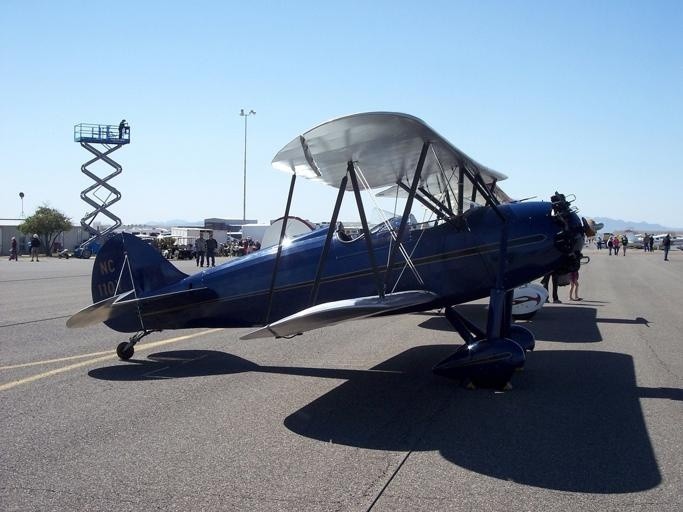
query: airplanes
[64,110,606,393]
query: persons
[611,237,620,256]
[596,236,601,251]
[160,238,261,262]
[567,271,583,302]
[606,236,612,256]
[7,236,17,261]
[648,234,654,252]
[621,234,628,256]
[204,234,217,267]
[336,223,353,241]
[643,233,649,252]
[194,233,206,268]
[541,273,562,303]
[29,234,40,262]
[662,234,671,261]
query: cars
[626,232,683,251]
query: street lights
[236,105,257,224]
